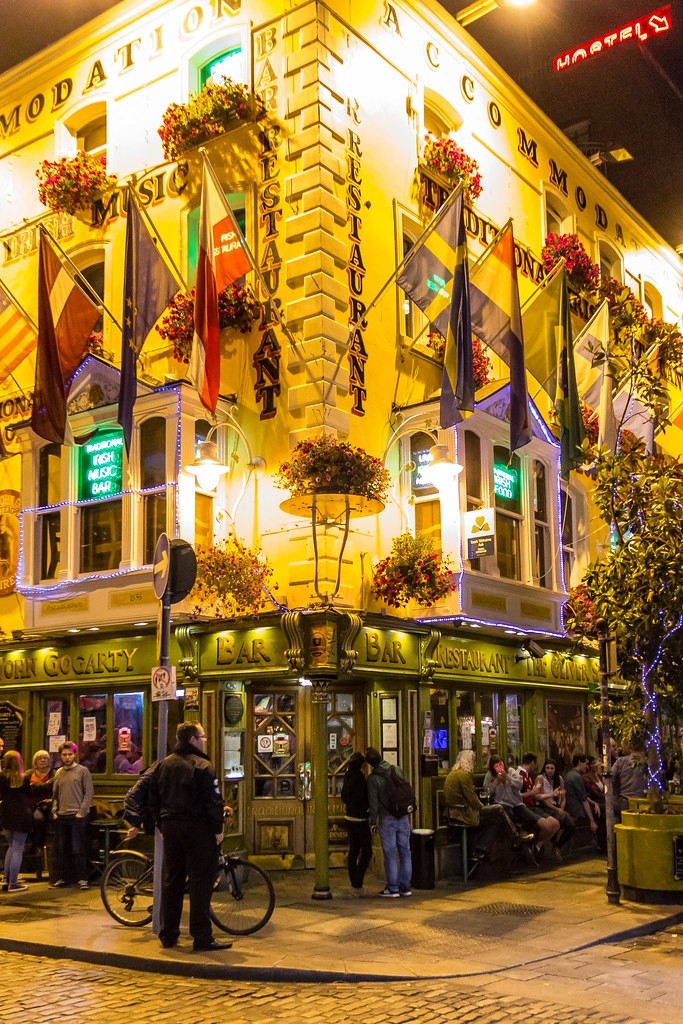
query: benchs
[513,820,597,868]
[444,804,483,883]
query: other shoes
[2,883,9,892]
[351,886,370,897]
[514,833,535,847]
[473,851,485,860]
[549,841,565,865]
[53,879,70,887]
[596,841,608,851]
[528,845,541,866]
[7,884,28,892]
[78,879,89,889]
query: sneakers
[378,886,400,898]
[400,888,412,896]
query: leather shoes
[198,941,232,950]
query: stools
[90,800,127,868]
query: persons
[122,720,234,952]
[0,734,5,882]
[0,749,35,892]
[48,741,94,891]
[23,750,57,880]
[79,721,143,774]
[441,736,649,867]
[341,752,373,897]
[365,747,417,898]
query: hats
[366,746,381,766]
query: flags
[0,156,257,460]
[394,186,667,552]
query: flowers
[271,431,394,504]
[423,129,483,204]
[547,396,676,468]
[35,148,119,212]
[186,532,272,623]
[74,278,253,364]
[427,330,495,394]
[157,73,249,163]
[368,531,456,610]
[542,231,683,372]
[562,582,594,636]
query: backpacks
[373,766,417,820]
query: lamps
[589,144,635,167]
[184,405,289,612]
[371,404,463,615]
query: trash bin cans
[88,819,125,880]
[411,827,435,890]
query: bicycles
[101,810,277,936]
